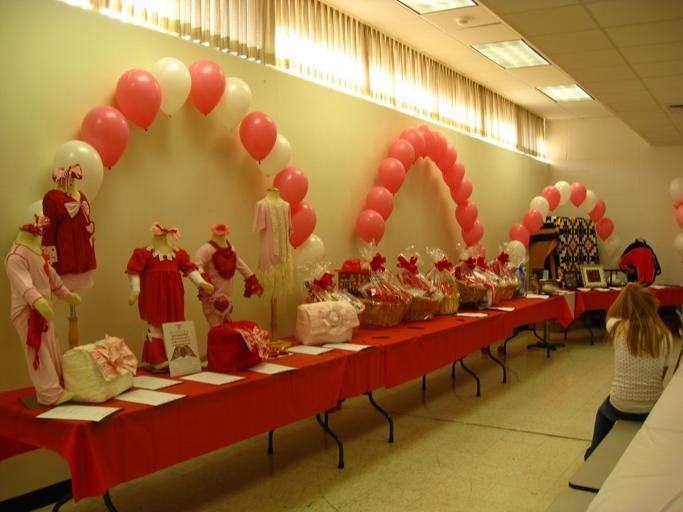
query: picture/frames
[562,264,608,291]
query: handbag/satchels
[297,301,359,345]
[208,321,270,374]
[63,337,133,402]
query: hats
[335,259,361,273]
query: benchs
[565,417,645,495]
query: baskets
[354,273,412,327]
[404,271,444,322]
[426,266,460,315]
[456,262,518,303]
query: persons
[1,224,84,406]
[123,225,213,370]
[615,237,662,284]
[584,283,674,460]
[34,168,100,292]
[189,225,262,328]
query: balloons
[505,181,614,268]
[669,177,682,258]
[112,68,156,133]
[255,132,325,271]
[29,198,48,230]
[356,123,485,260]
[148,57,195,120]
[79,105,127,174]
[182,59,226,115]
[214,76,250,132]
[240,111,278,163]
[45,139,104,209]
